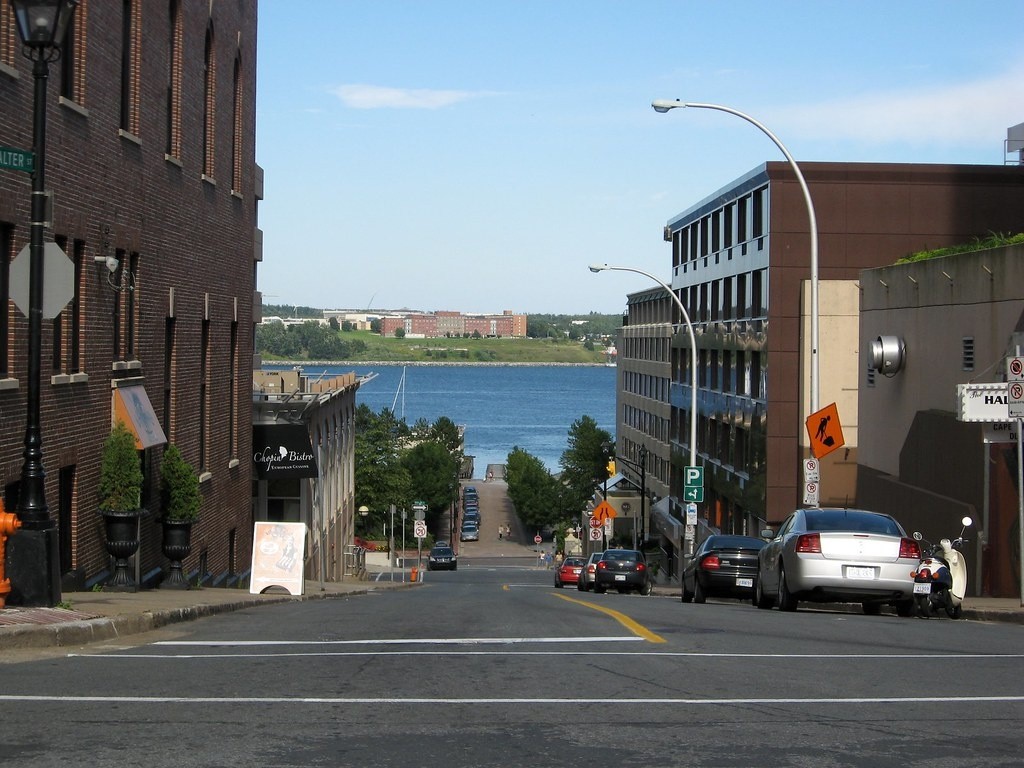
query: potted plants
[156,440,202,592]
[91,424,149,592]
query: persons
[499,525,504,541]
[506,524,511,536]
[540,549,565,570]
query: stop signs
[589,516,602,528]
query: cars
[755,507,923,618]
[460,486,482,542]
[554,554,588,588]
[426,541,459,572]
[594,548,652,596]
[680,533,769,607]
[577,552,604,592]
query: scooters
[911,515,973,622]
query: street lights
[587,261,698,564]
[650,96,822,507]
[8,1,78,532]
